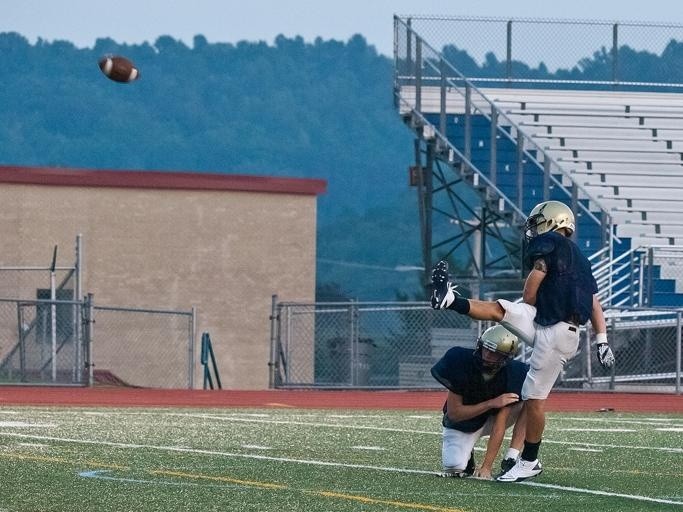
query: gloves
[597,342,616,373]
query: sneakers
[430,259,462,311]
[463,447,476,478]
[494,457,544,483]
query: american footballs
[100,57,140,82]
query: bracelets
[596,333,608,344]
[504,448,517,460]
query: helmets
[524,200,576,245]
[473,324,519,370]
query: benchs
[400,79,681,320]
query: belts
[563,319,580,327]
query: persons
[430,323,532,481]
[430,201,614,484]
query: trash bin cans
[327,336,377,387]
